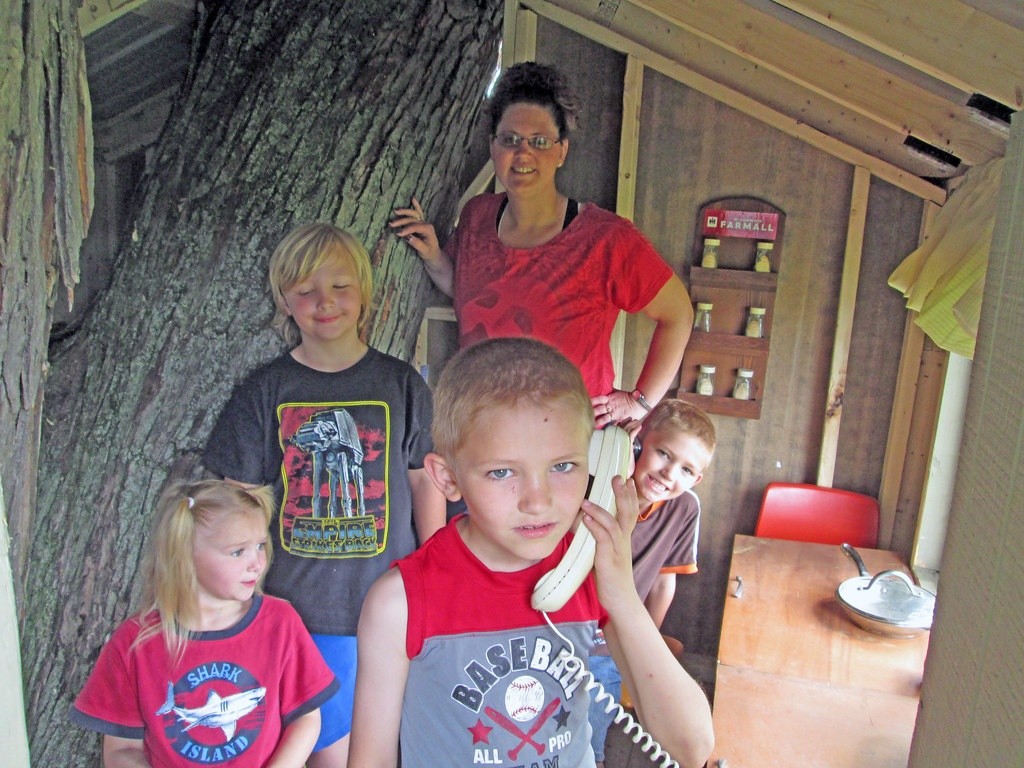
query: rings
[417,216,424,222]
[610,413,615,422]
[604,404,611,413]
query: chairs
[756,481,880,550]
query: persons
[198,221,447,767]
[70,478,341,767]
[387,59,694,520]
[629,399,720,629]
[345,338,715,768]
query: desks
[701,534,931,768]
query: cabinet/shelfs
[676,197,786,418]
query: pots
[834,543,936,639]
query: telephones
[530,421,630,611]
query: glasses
[495,132,559,150]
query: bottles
[745,307,766,338]
[733,368,753,400]
[701,239,720,268]
[696,365,716,396]
[693,302,714,333]
[756,242,774,273]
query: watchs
[631,389,654,414]
